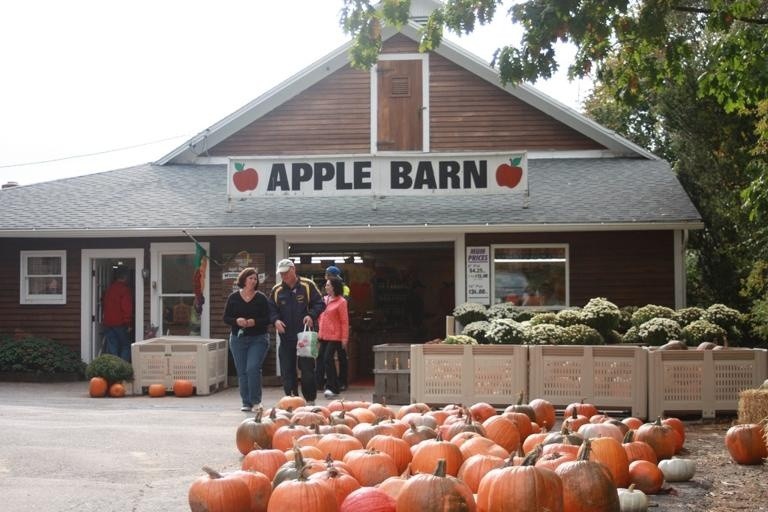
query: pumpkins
[149,384,166,397]
[173,381,192,396]
[725,424,768,464]
[188,396,696,512]
[110,384,124,398]
[89,377,107,398]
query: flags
[190,241,208,316]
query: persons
[98,264,134,364]
[315,266,351,391]
[538,279,560,306]
[268,258,326,406]
[223,266,273,415]
[523,284,539,306]
[318,278,349,400]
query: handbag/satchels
[296,324,319,359]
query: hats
[326,265,341,275]
[276,258,294,274]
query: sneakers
[324,389,338,398]
[251,403,264,413]
[305,400,317,406]
[241,403,251,412]
